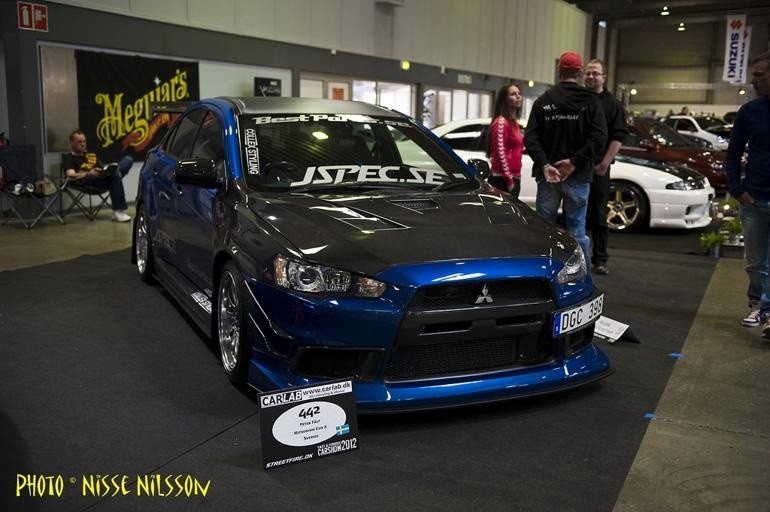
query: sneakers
[110,211,132,224]
[739,306,770,339]
[595,264,610,276]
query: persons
[726,53,770,340]
[562,58,629,274]
[62,130,133,222]
[522,51,609,270]
[646,106,715,121]
[486,84,524,197]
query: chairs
[0,144,68,230]
[58,151,115,222]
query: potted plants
[698,196,743,259]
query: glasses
[584,71,606,77]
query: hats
[558,51,585,71]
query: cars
[132,96,616,414]
[618,118,743,197]
[660,115,750,166]
[357,118,713,231]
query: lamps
[312,122,329,141]
